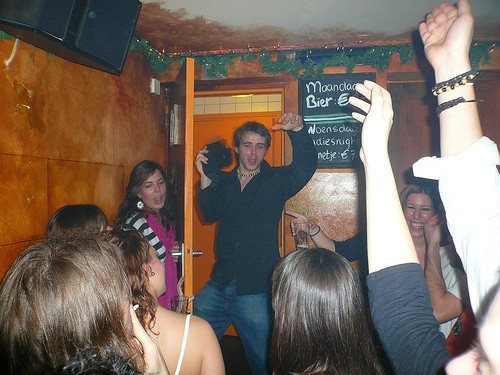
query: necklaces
[237,165,261,177]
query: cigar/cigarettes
[132,305,140,312]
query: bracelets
[309,225,321,236]
[432,69,481,117]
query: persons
[116,159,181,313]
[398,180,473,341]
[284,210,365,266]
[1,237,148,375]
[409,0,500,374]
[191,111,318,375]
[99,230,225,375]
[47,203,113,245]
[266,248,386,375]
[349,79,500,375]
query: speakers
[0,0,142,76]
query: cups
[168,296,190,314]
[294,219,310,250]
